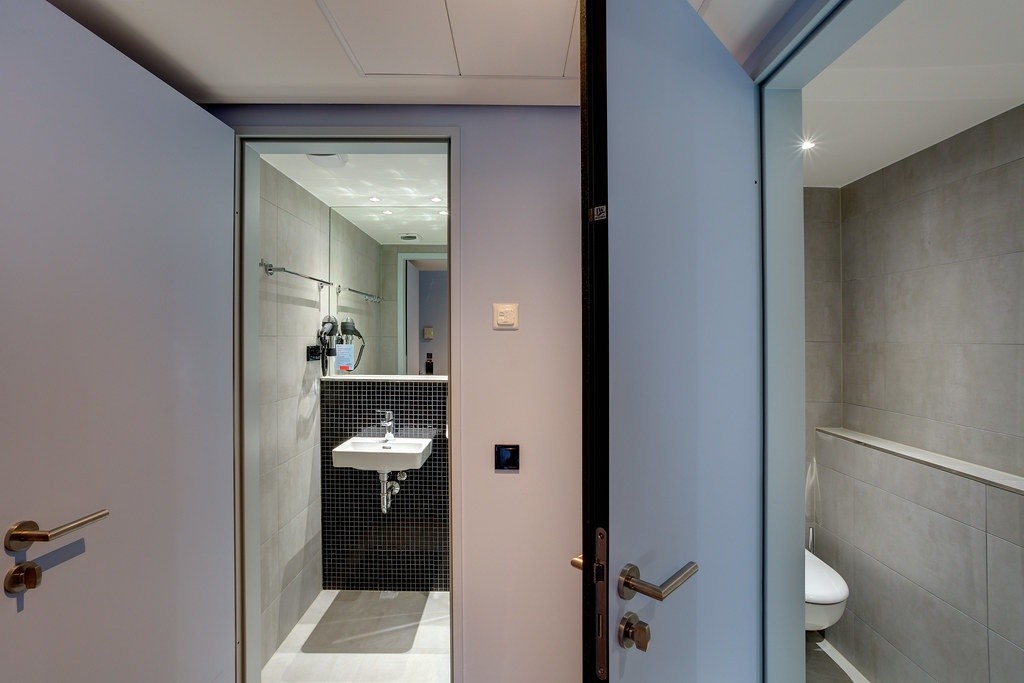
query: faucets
[374,408,396,439]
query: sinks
[332,436,434,472]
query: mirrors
[328,205,450,377]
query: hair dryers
[319,314,339,358]
[340,315,365,350]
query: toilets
[805,534,851,633]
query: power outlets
[492,302,522,333]
[421,326,436,340]
